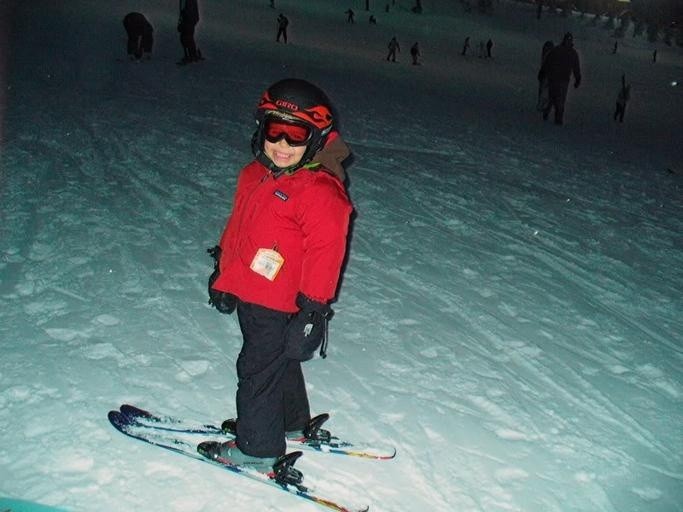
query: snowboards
[537,41,555,111]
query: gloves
[204,245,237,314]
[284,292,333,362]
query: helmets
[253,77,334,173]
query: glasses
[260,117,315,147]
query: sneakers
[235,415,311,442]
[220,439,282,472]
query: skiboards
[108,404,396,512]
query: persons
[173,0,202,68]
[119,9,155,62]
[610,83,632,123]
[191,78,358,477]
[265,1,675,67]
[536,31,583,128]
